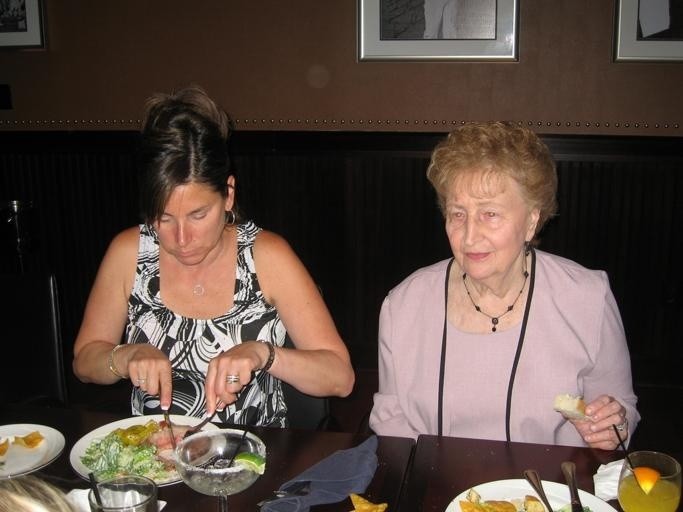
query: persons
[70,84,356,430]
[365,118,643,452]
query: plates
[70,414,227,486]
[444,478,615,512]
[0,424,67,479]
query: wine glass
[173,427,269,511]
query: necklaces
[171,256,234,298]
[461,244,530,334]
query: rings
[224,374,239,384]
[609,416,628,433]
[135,377,146,386]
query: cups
[617,450,682,512]
[88,475,159,512]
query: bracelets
[107,342,129,381]
[256,337,275,372]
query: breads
[459,496,545,512]
[552,394,586,419]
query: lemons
[632,466,661,495]
[233,451,267,476]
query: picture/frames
[0,0,50,55]
[354,0,523,67]
[608,0,682,67]
